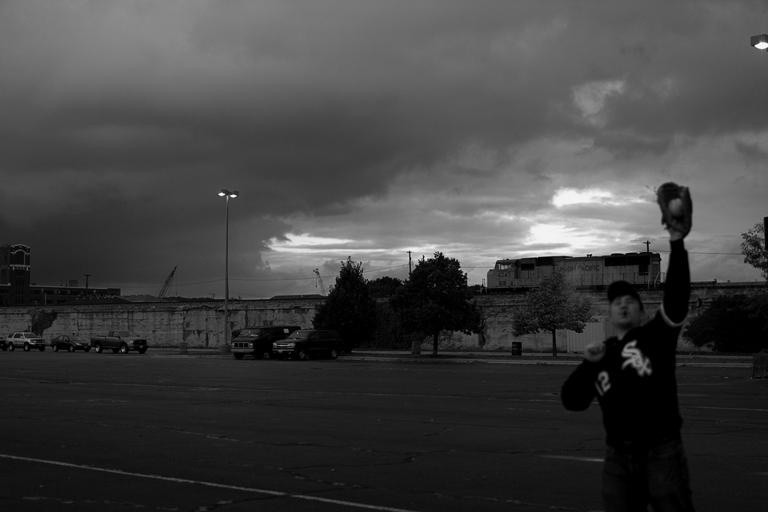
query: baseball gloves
[657,182,691,234]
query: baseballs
[668,198,681,216]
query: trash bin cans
[412,340,420,354]
[512,342,522,357]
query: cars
[231,326,341,360]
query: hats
[606,280,644,313]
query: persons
[560,182,700,511]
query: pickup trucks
[0,331,148,354]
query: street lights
[217,188,239,346]
